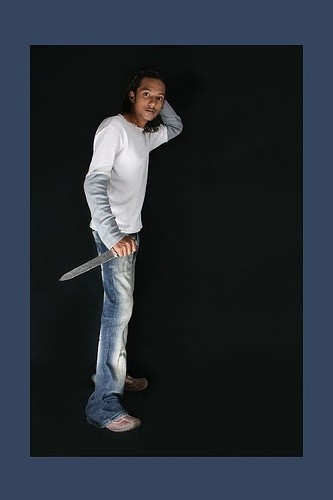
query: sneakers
[106,414,142,433]
[92,374,148,391]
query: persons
[84,71,184,431]
